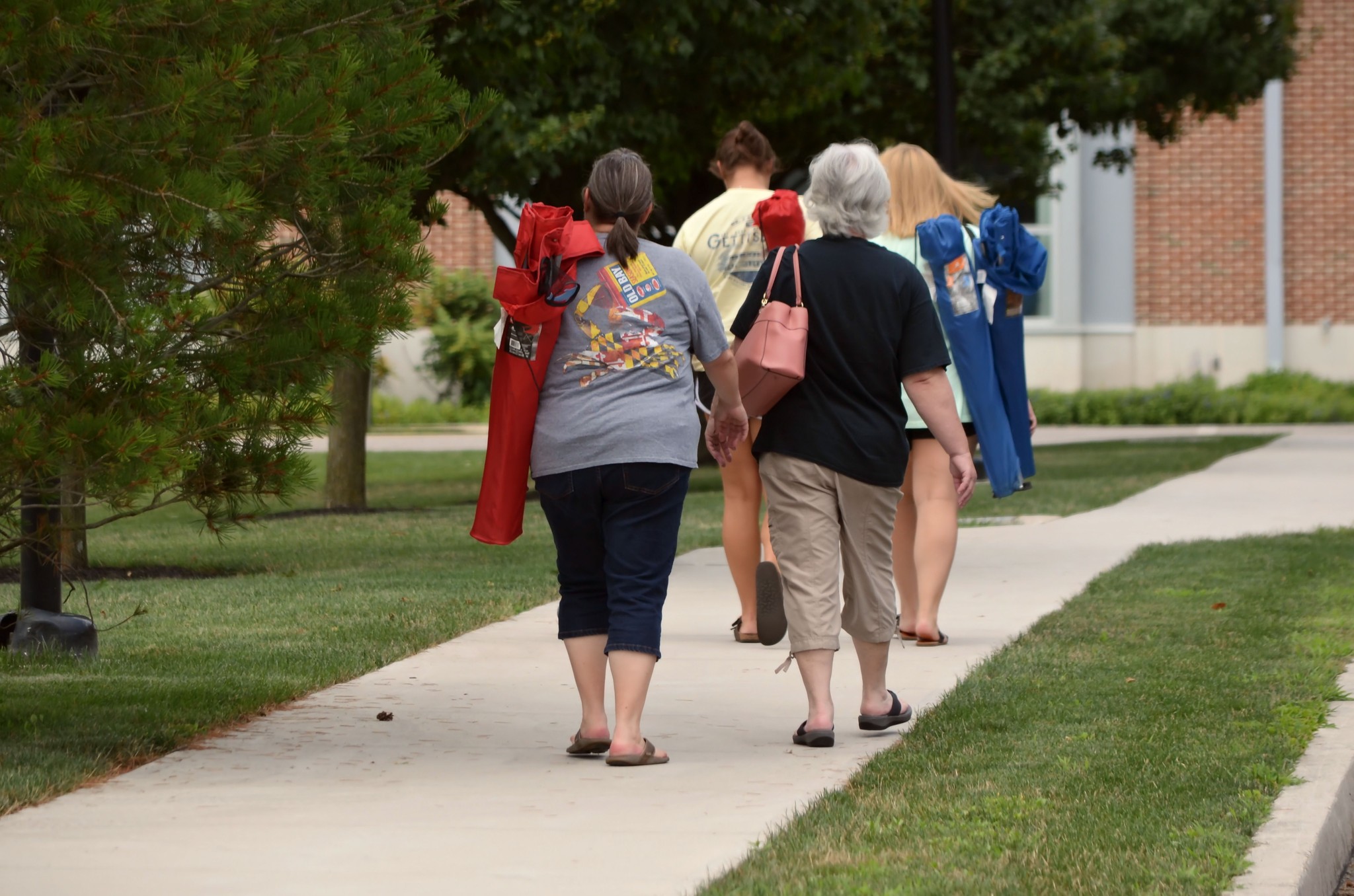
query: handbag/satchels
[735,243,808,418]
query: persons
[669,119,804,642]
[703,142,978,748]
[492,149,749,769]
[866,143,1036,648]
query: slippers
[604,735,670,764]
[916,629,949,647]
[894,614,917,640]
[567,729,612,755]
[730,614,760,643]
[859,689,913,731]
[756,560,786,647]
[792,719,835,748]
